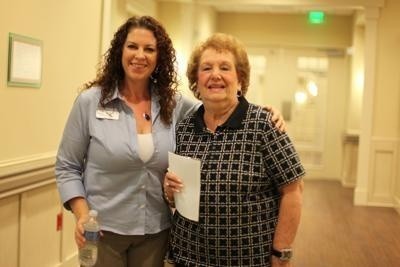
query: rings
[166,182,170,187]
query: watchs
[271,249,293,260]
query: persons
[54,14,288,267]
[162,32,307,266]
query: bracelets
[163,189,175,208]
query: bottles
[78,210,100,266]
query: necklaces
[128,91,151,122]
